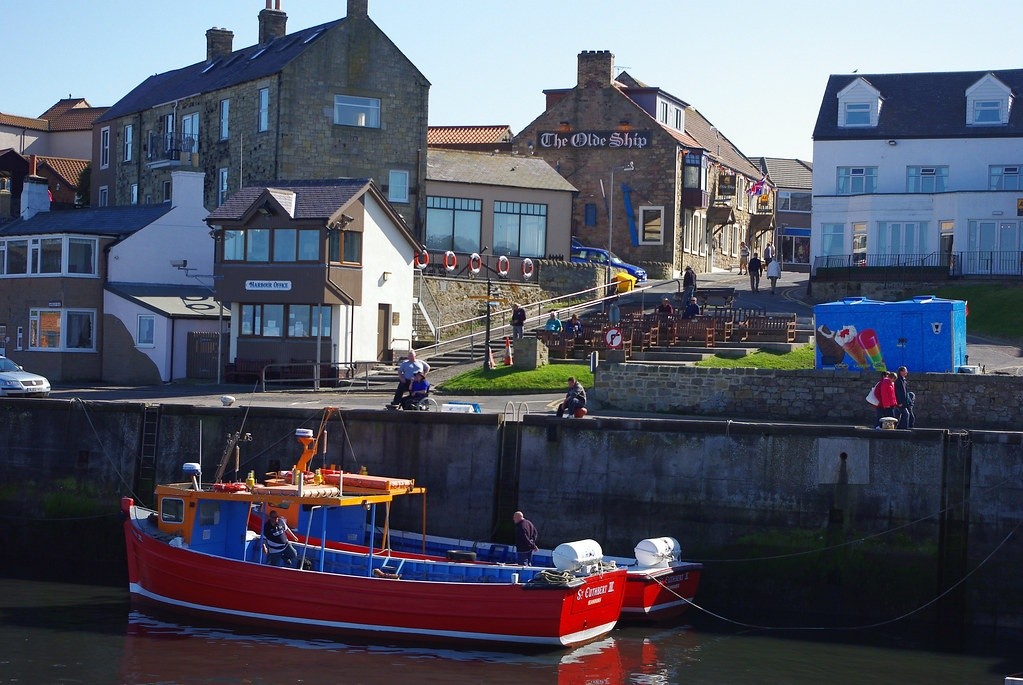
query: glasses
[691,300,695,301]
[272,516,279,519]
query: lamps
[620,121,629,125]
[889,141,896,145]
[334,214,354,230]
[560,122,568,125]
[257,208,271,215]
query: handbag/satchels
[866,382,881,406]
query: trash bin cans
[225,363,236,382]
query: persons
[556,377,587,419]
[391,348,430,409]
[766,257,781,295]
[738,242,750,275]
[764,241,776,271]
[564,314,583,339]
[656,266,701,322]
[545,312,563,336]
[871,365,915,429]
[513,511,539,566]
[263,510,298,567]
[509,303,527,339]
[748,253,763,293]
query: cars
[570,246,648,286]
[0,355,53,400]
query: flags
[744,174,768,197]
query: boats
[366,532,707,630]
[119,407,627,651]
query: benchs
[533,288,797,360]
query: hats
[408,351,416,355]
[413,371,425,379]
[663,298,669,301]
[572,314,579,320]
[685,266,691,270]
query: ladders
[380,558,405,574]
[486,545,508,563]
[139,407,158,480]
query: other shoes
[391,401,399,405]
[282,555,292,565]
[567,415,573,419]
[738,272,748,275]
